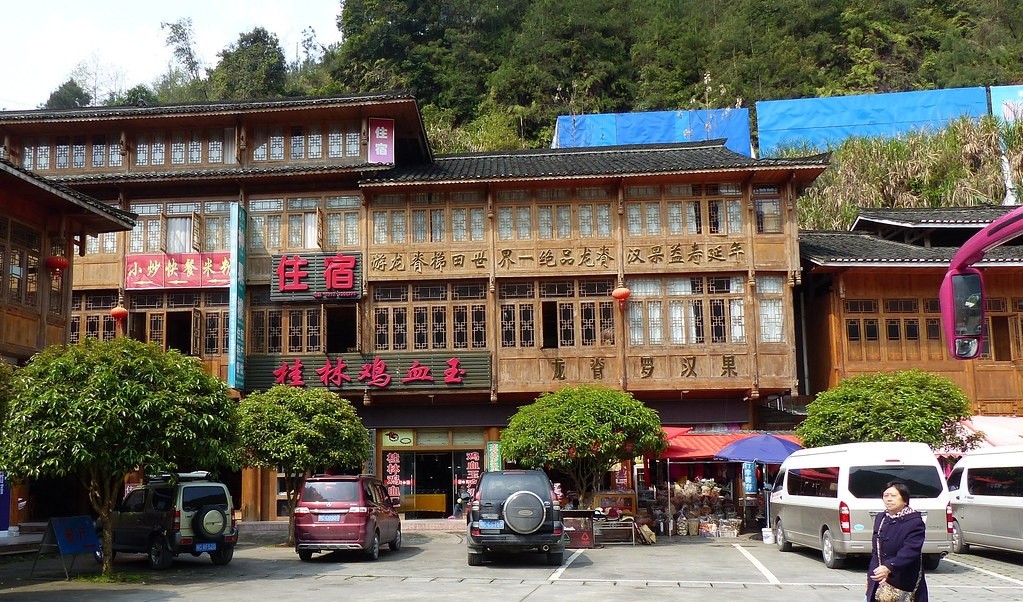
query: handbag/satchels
[875,581,915,602]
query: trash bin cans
[0,469,11,531]
[560,509,595,549]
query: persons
[866,482,928,602]
[454,498,463,518]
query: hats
[593,507,635,523]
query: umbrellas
[715,434,805,525]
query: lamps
[787,200,793,211]
[487,209,495,219]
[617,205,624,216]
[747,201,755,211]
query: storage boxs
[699,529,719,537]
[720,531,737,538]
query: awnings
[646,434,809,523]
[930,416,1023,458]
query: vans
[946,452,1023,554]
[763,442,952,571]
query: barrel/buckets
[762,528,776,545]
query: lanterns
[48,256,68,275]
[612,287,630,308]
[112,308,128,326]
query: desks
[593,521,635,546]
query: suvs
[294,473,401,560]
[94,471,239,570]
[467,469,564,566]
